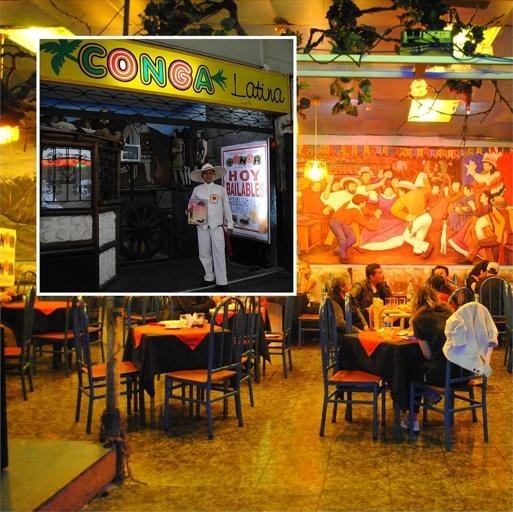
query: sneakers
[400,416,419,432]
[424,390,442,409]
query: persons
[327,259,508,434]
[300,151,513,267]
[86,295,217,346]
[184,162,235,288]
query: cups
[385,297,405,307]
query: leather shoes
[215,285,231,289]
[197,281,214,287]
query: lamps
[409,78,428,98]
[304,96,329,182]
[0,111,24,146]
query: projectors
[401,30,453,46]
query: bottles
[384,313,392,340]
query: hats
[189,162,227,183]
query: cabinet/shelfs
[297,214,323,254]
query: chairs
[1,265,513,450]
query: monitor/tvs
[120,144,141,163]
